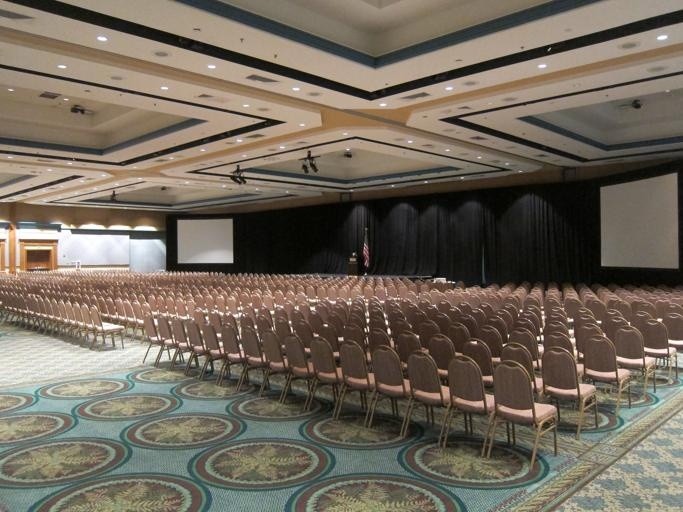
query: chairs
[142,298,374,427]
[0,265,460,352]
[369,280,683,472]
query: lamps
[228,163,246,186]
[300,149,320,174]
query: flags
[362,243,370,268]
